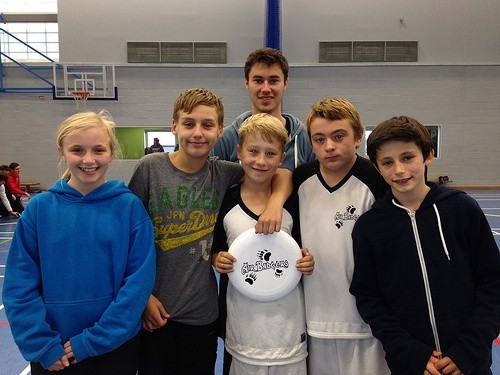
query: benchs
[19,182,40,193]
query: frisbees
[227,227,303,302]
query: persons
[208,48,317,171]
[349,116,500,375]
[145,138,164,155]
[2,112,157,375]
[0,162,31,217]
[128,88,292,375]
[212,113,315,375]
[292,97,390,375]
